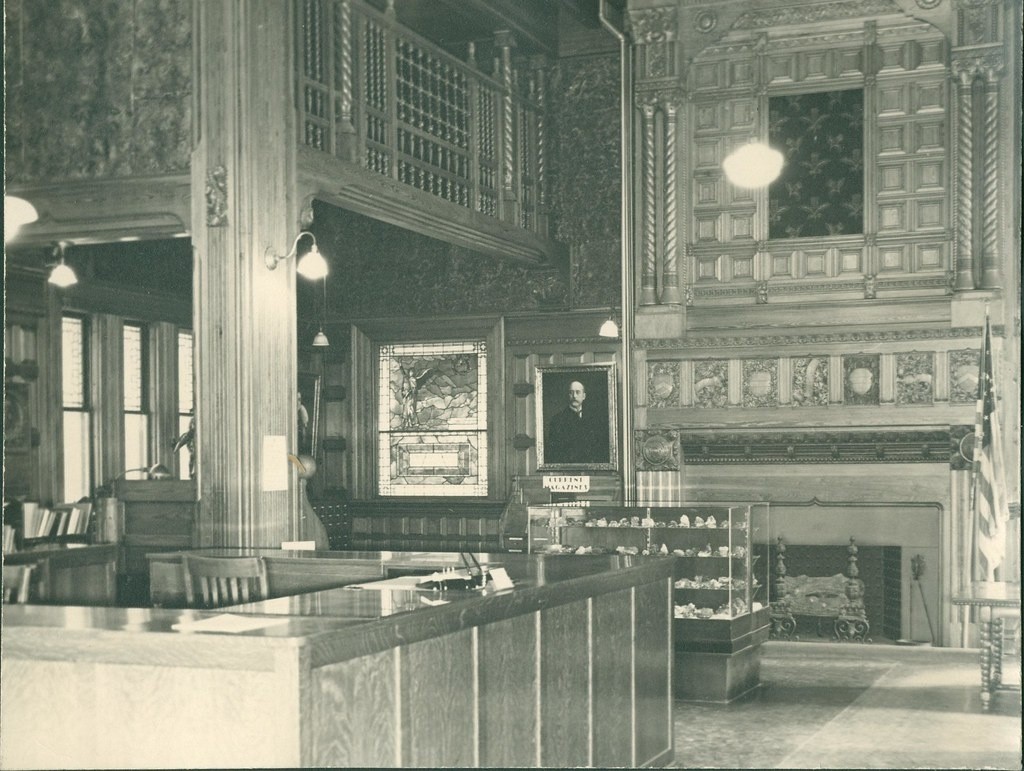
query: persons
[549,380,607,461]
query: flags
[971,317,1010,580]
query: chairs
[178,551,270,611]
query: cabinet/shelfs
[2,538,121,606]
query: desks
[951,578,1022,713]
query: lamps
[115,464,170,481]
[264,229,328,282]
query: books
[5,501,92,554]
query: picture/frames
[533,363,619,470]
